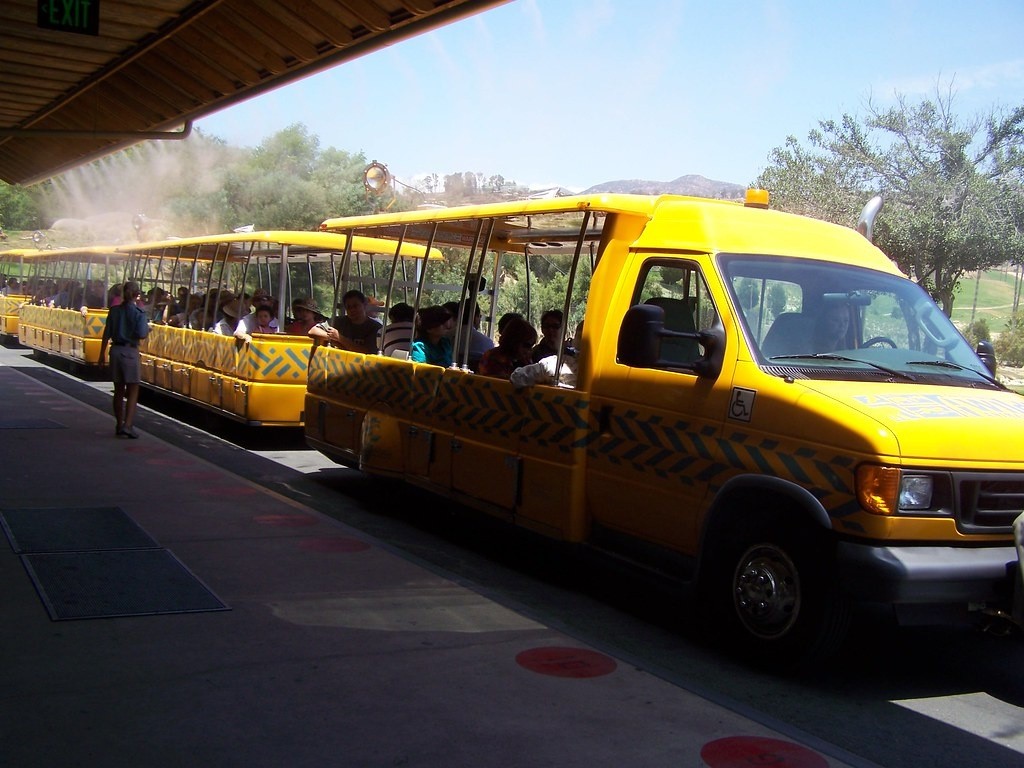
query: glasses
[541,323,561,330]
[522,341,532,348]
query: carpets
[19,548,233,621]
[0,504,161,555]
[0,417,70,429]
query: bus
[1,188,1024,670]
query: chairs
[239,311,279,336]
[762,312,815,353]
[153,296,185,320]
[643,296,699,372]
[187,307,223,328]
[377,322,417,355]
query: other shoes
[116,424,139,439]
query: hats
[252,288,274,301]
[421,307,453,329]
[223,300,249,317]
[296,298,321,314]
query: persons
[479,308,584,388]
[813,301,854,353]
[98,282,154,439]
[1,274,329,343]
[376,298,495,376]
[307,289,383,355]
